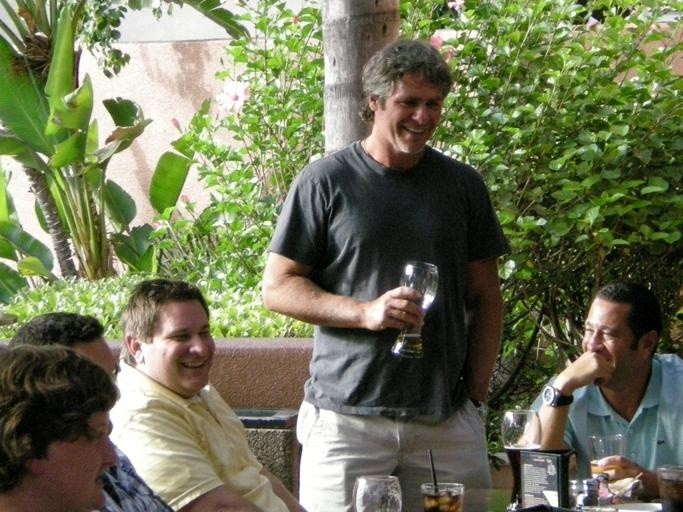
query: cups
[352,476,464,512]
[657,465,683,512]
[588,434,628,478]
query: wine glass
[502,409,542,512]
[391,259,439,361]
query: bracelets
[469,399,488,414]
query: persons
[5,312,176,511]
[521,282,683,502]
[0,340,121,511]
[108,277,307,512]
[260,39,513,512]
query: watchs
[540,384,574,407]
